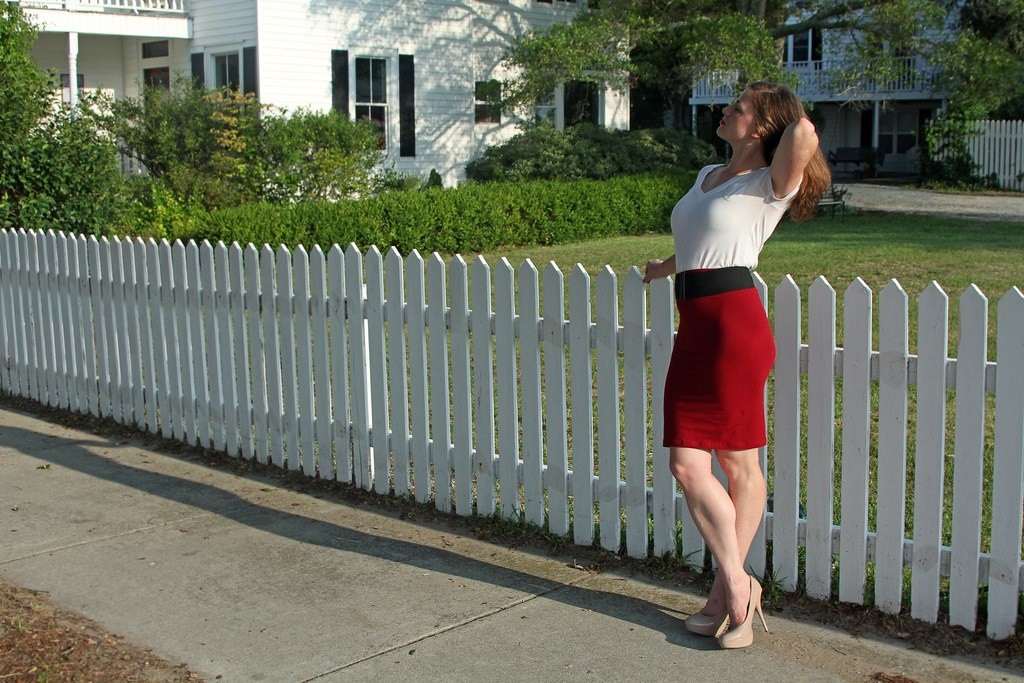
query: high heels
[717,573,768,649]
[684,607,730,637]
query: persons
[638,80,822,652]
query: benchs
[825,146,885,168]
